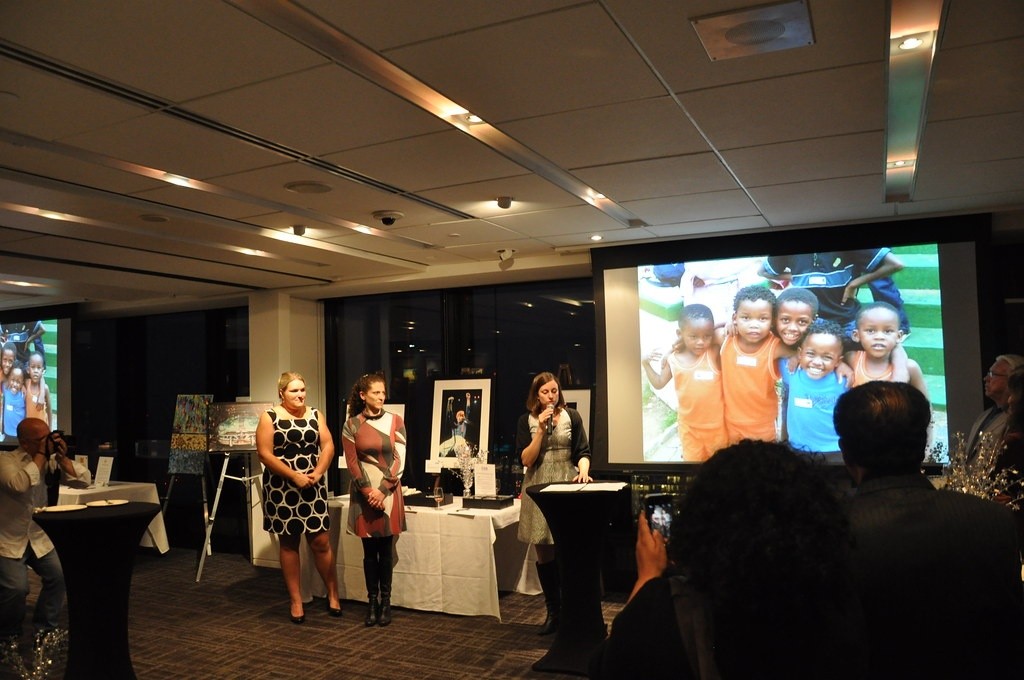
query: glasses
[987,370,1007,379]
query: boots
[365,594,377,626]
[378,598,391,626]
[535,559,559,636]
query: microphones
[546,404,555,435]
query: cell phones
[644,493,675,548]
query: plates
[42,505,88,511]
[86,499,130,506]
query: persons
[439,392,471,458]
[0,418,91,643]
[962,354,1023,486]
[598,439,913,680]
[992,364,1024,561]
[341,373,406,625]
[643,246,933,467]
[517,371,593,635]
[834,380,1024,680]
[0,321,53,438]
[255,371,342,622]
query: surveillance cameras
[499,249,513,261]
[372,210,403,225]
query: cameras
[50,431,76,453]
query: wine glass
[434,487,444,510]
[496,479,501,496]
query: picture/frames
[167,394,214,476]
[207,402,275,453]
[429,379,492,465]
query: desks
[56,481,170,554]
[527,478,629,675]
[330,492,520,619]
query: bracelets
[37,451,46,455]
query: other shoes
[290,604,305,624]
[327,594,343,616]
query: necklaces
[361,408,386,420]
